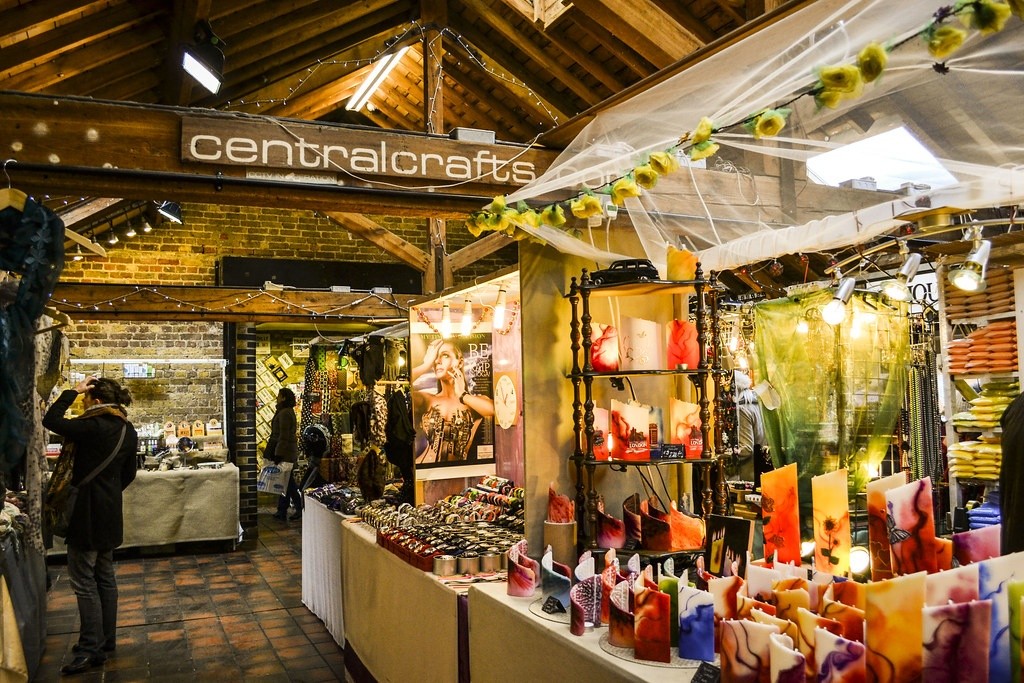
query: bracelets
[360,474,525,558]
[460,391,468,404]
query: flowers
[465,0,1024,249]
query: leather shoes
[72,644,116,653]
[62,656,107,674]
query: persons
[998,392,1024,553]
[413,339,495,464]
[263,388,304,520]
[42,376,138,674]
[735,371,774,490]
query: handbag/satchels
[263,439,277,460]
[49,486,79,538]
[257,458,294,497]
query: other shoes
[288,510,302,520]
[268,514,287,523]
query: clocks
[493,370,518,430]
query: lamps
[179,17,228,96]
[91,235,101,246]
[108,227,119,244]
[435,278,509,337]
[141,215,152,233]
[72,243,84,261]
[820,277,857,325]
[947,239,992,292]
[345,46,410,112]
[126,221,136,237]
[157,201,182,224]
[880,253,923,302]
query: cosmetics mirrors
[176,436,197,468]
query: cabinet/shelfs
[934,240,1024,539]
[42,430,244,557]
[563,262,730,576]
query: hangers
[0,272,73,335]
[0,159,106,258]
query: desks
[341,518,508,683]
[468,580,721,683]
[302,491,361,649]
